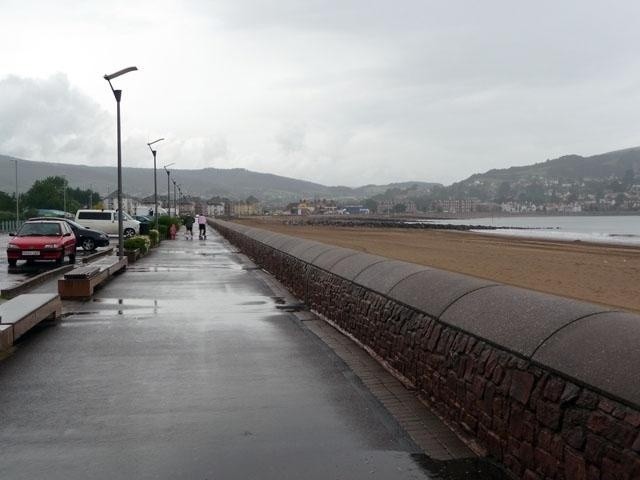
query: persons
[169,223,177,239]
[183,214,195,236]
[198,213,207,237]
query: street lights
[146,138,165,230]
[63,174,67,212]
[164,162,183,217]
[103,66,138,256]
[10,158,19,229]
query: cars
[6,216,109,265]
[131,216,151,222]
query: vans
[75,209,142,239]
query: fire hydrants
[170,223,177,240]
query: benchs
[0,294,60,354]
[58,256,128,302]
